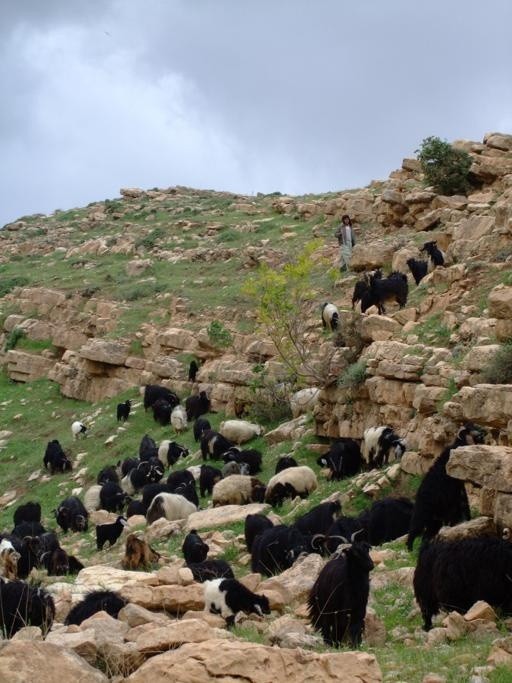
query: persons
[333,213,358,274]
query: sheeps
[187,360,198,382]
[420,240,454,271]
[406,258,427,286]
[0,383,512,651]
[350,268,408,316]
[321,302,340,333]
